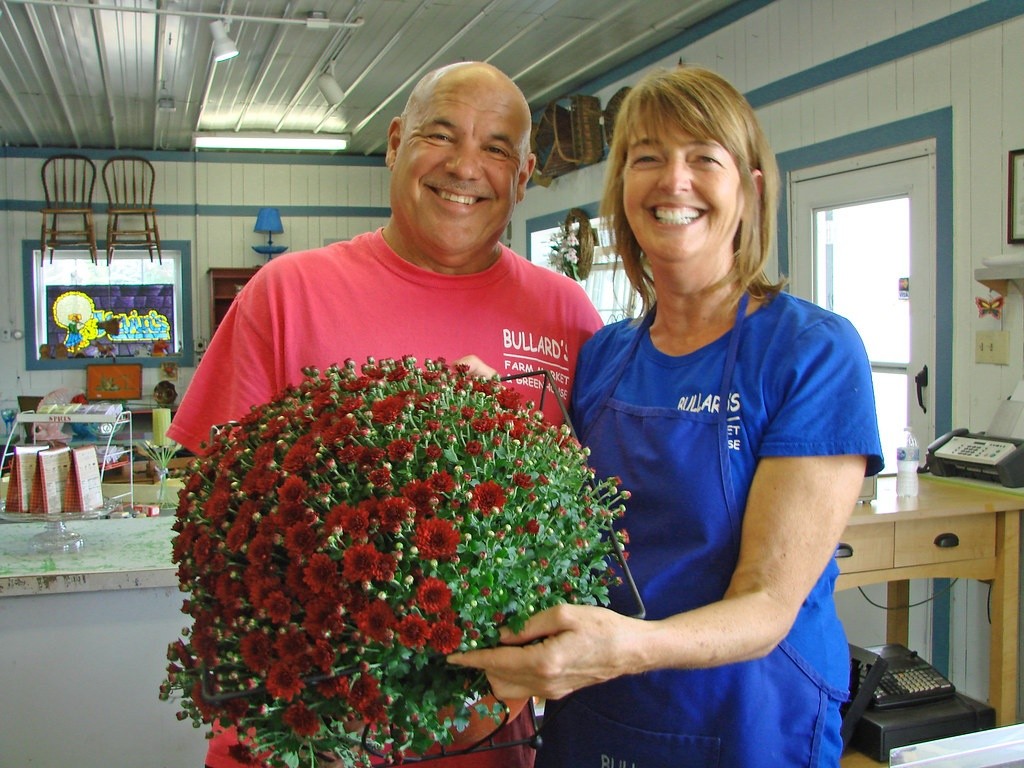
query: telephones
[926,428,1024,486]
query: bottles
[896,427,920,498]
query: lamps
[192,133,351,152]
[210,17,239,62]
[252,206,288,263]
[152,408,171,480]
[156,80,176,113]
[317,58,345,105]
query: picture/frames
[1008,149,1023,245]
[86,364,142,400]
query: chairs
[17,396,65,448]
[40,154,97,267]
[101,157,162,266]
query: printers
[924,399,1024,488]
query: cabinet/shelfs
[206,267,263,342]
[114,405,197,477]
[0,410,134,510]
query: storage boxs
[133,505,159,516]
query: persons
[444,66,885,768]
[160,60,612,768]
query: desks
[0,517,210,768]
[831,474,1023,768]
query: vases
[392,694,532,752]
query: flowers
[547,222,583,286]
[158,355,631,768]
